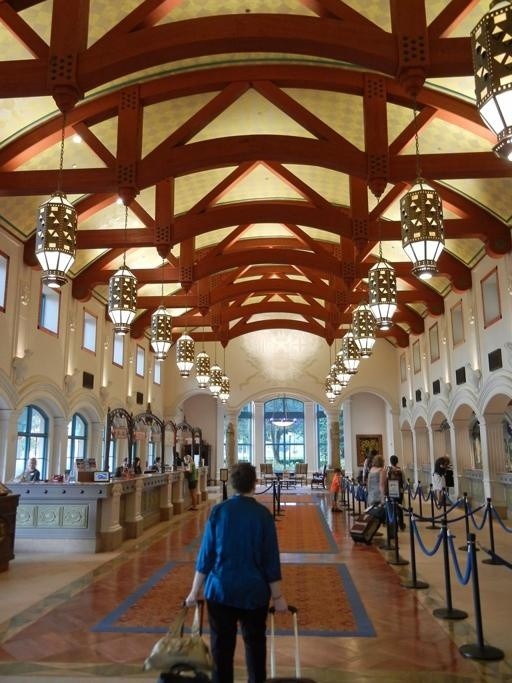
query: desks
[273,480,297,490]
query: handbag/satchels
[144,634,214,670]
[368,505,386,523]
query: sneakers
[332,508,343,512]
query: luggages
[157,600,211,683]
[350,503,385,544]
[267,605,313,683]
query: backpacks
[389,466,404,493]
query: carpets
[184,496,338,554]
[91,560,377,637]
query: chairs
[288,464,308,487]
[259,464,277,487]
[311,464,326,490]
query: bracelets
[271,593,282,600]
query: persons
[178,455,198,511]
[115,452,182,477]
[186,463,288,682]
[433,456,450,506]
[329,468,341,512]
[16,458,41,483]
[362,448,406,536]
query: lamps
[342,321,360,375]
[196,312,210,390]
[209,336,221,398]
[326,345,336,402]
[470,0,512,165]
[35,97,78,291]
[335,348,352,388]
[177,286,195,379]
[153,251,172,358]
[400,92,445,282]
[270,393,297,426]
[368,196,396,331]
[331,338,342,394]
[354,280,376,360]
[219,348,229,404]
[109,204,138,335]
